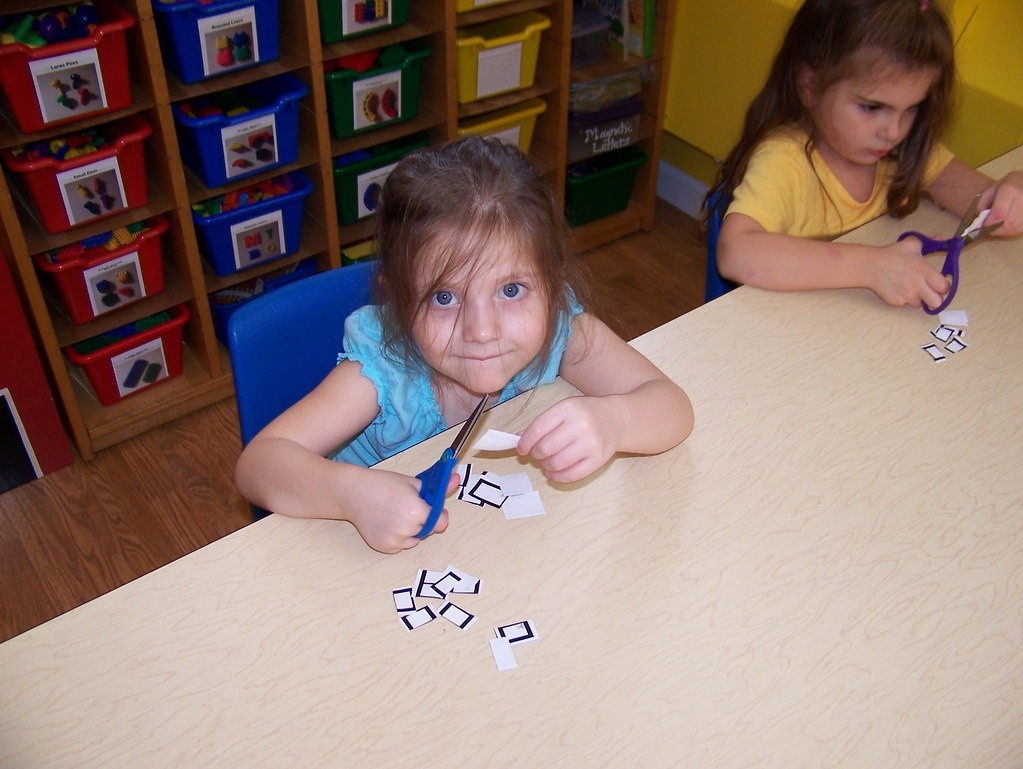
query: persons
[237,136,695,554]
[700,0,1023,310]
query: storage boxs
[565,148,648,227]
[1,0,553,406]
[571,8,612,70]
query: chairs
[228,260,383,524]
[706,185,735,301]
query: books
[582,0,656,64]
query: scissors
[415,393,488,539]
[896,194,1004,316]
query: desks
[0,144,1022,768]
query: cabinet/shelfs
[0,0,675,462]
